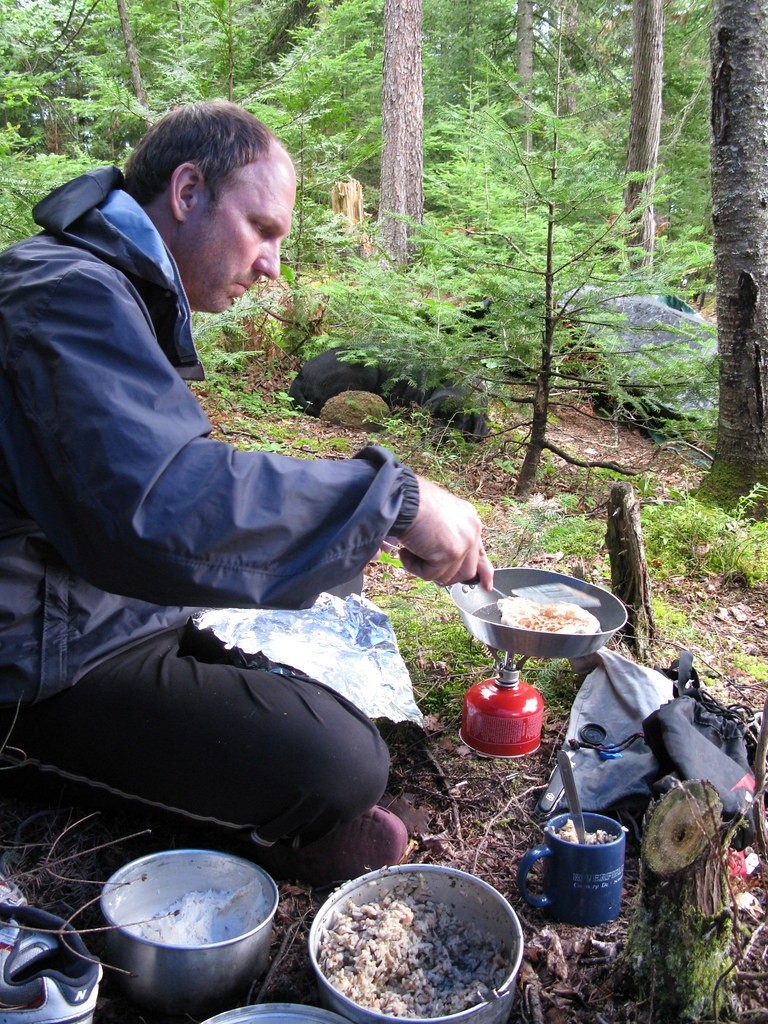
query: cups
[516,813,626,927]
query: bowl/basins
[99,850,281,1022]
[307,863,525,1024]
[198,1003,356,1024]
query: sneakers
[296,805,409,890]
[0,873,104,1024]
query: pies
[495,595,601,638]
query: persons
[2,109,498,887]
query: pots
[445,567,628,659]
